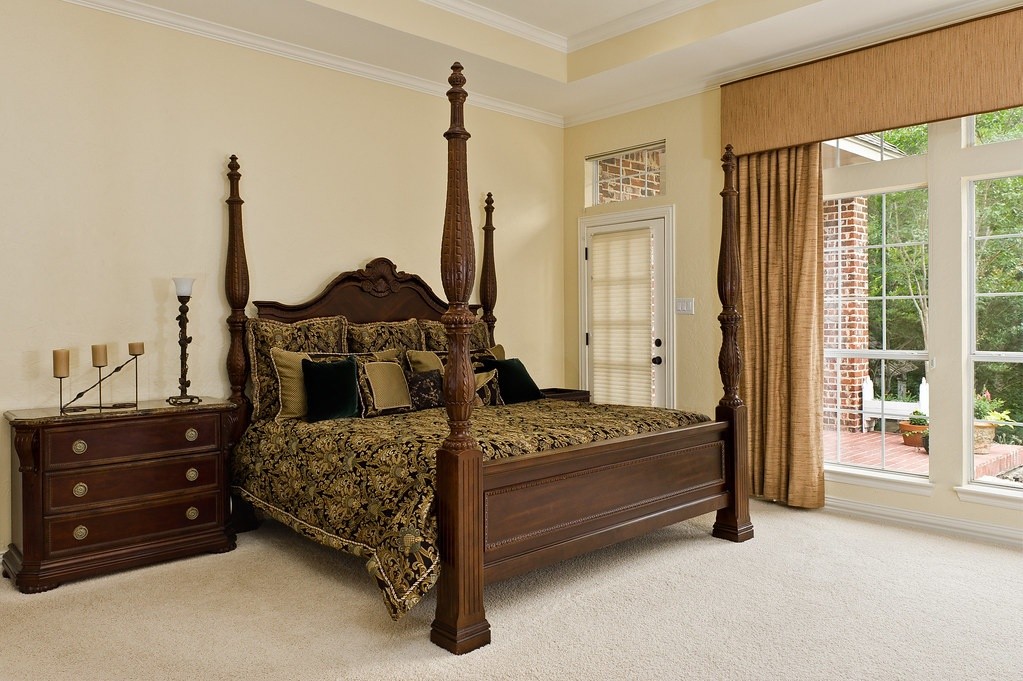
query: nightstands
[4,406,240,596]
[538,387,590,403]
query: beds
[228,61,752,656]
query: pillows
[242,315,546,427]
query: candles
[53,347,70,379]
[92,345,109,368]
[129,341,144,355]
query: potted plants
[972,396,996,454]
[899,413,930,453]
